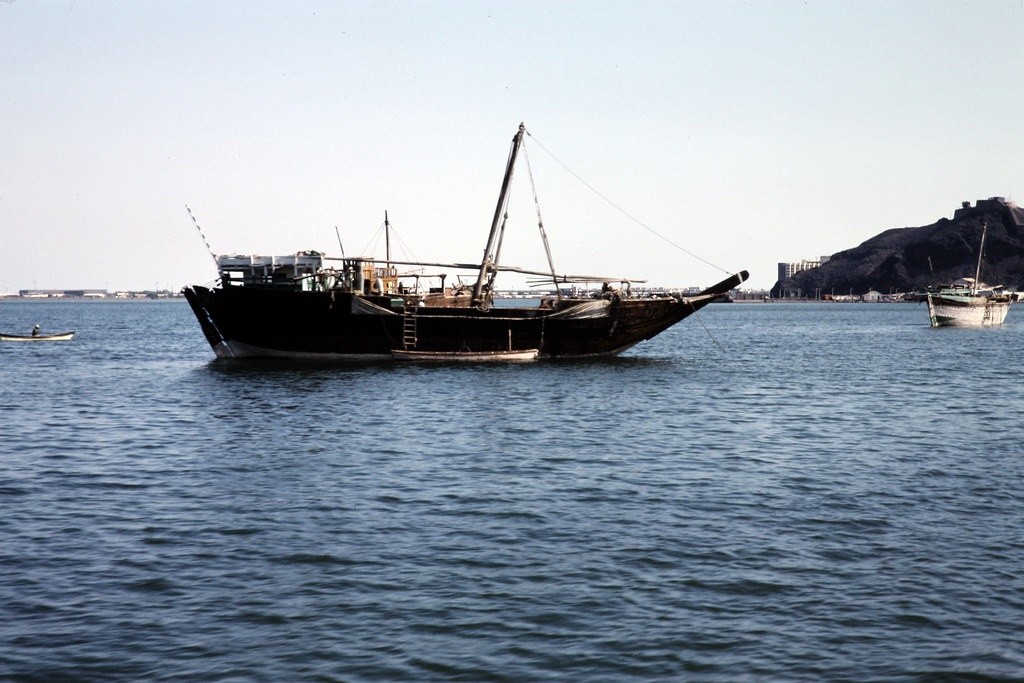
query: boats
[0,330,77,343]
[182,125,752,365]
[392,329,541,367]
[916,221,1013,328]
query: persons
[485,281,494,307]
[32,324,40,337]
[398,283,403,294]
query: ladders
[403,294,418,350]
